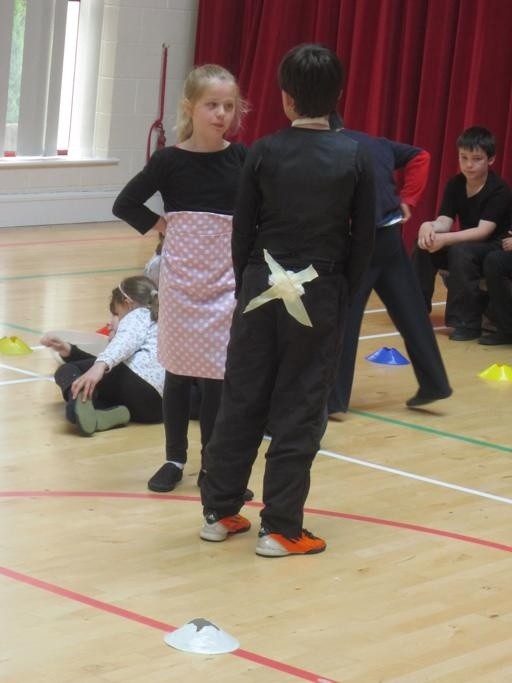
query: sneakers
[147,463,182,493]
[200,513,251,542]
[255,528,326,557]
[66,389,96,434]
[196,471,254,501]
[94,404,130,432]
[406,385,451,406]
[448,328,479,341]
[479,332,510,345]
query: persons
[477,236,512,347]
[195,42,376,558]
[39,315,122,362]
[111,61,255,501]
[328,107,455,421]
[410,124,510,341]
[54,274,167,434]
